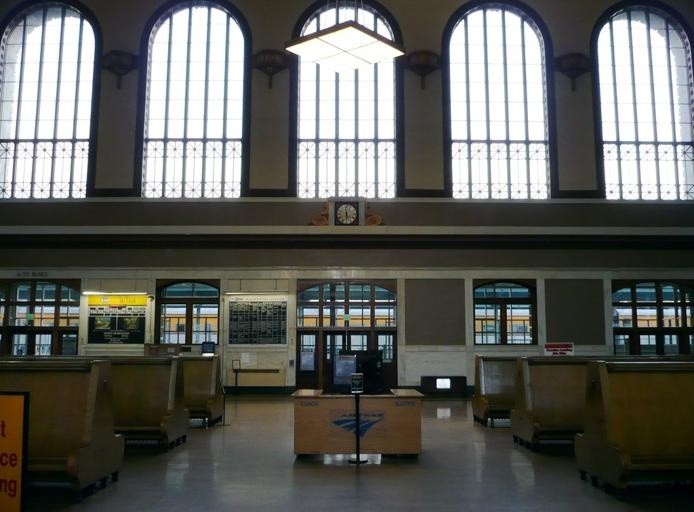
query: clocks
[329,194,363,226]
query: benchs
[90,356,188,455]
[0,359,126,502]
[179,356,227,430]
[508,354,587,454]
[470,354,522,426]
[570,360,692,502]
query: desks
[290,387,423,461]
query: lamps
[284,1,404,75]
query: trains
[0,299,692,358]
